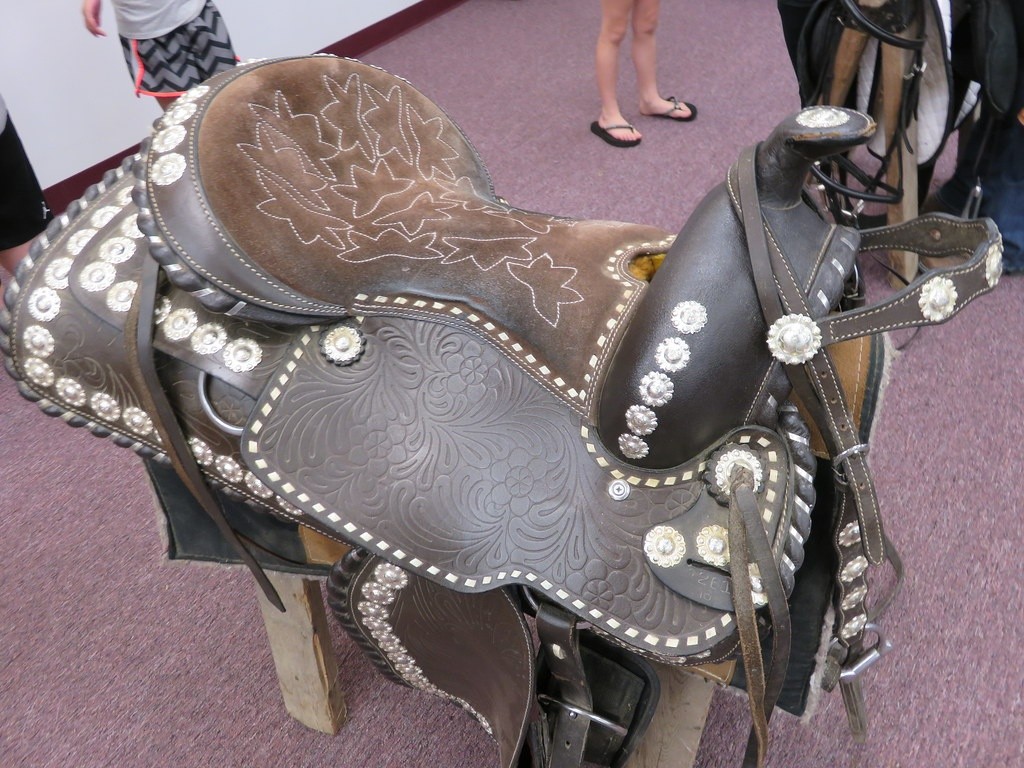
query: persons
[916,0,1024,281]
[83,0,239,113]
[591,0,697,146]
[0,92,55,311]
